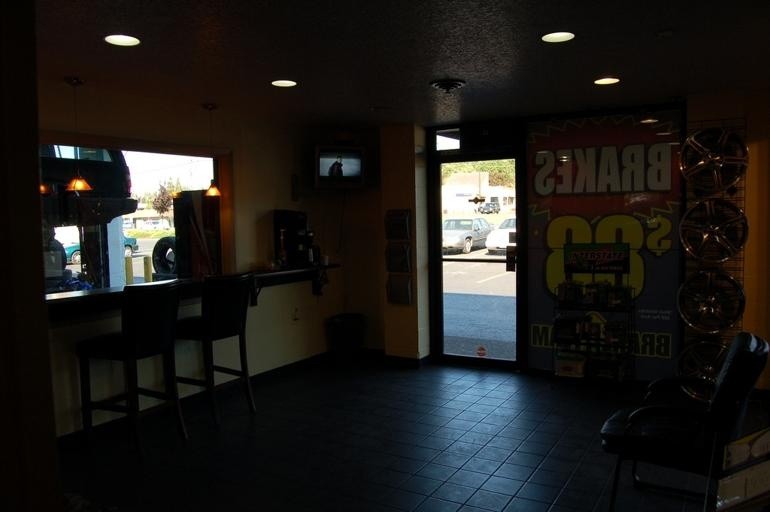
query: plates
[550,285,636,383]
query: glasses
[62,75,94,197]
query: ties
[314,149,366,188]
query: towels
[325,311,368,375]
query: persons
[328,156,343,177]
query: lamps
[76,270,258,463]
[600,331,769,511]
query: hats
[484,218,516,256]
[48,231,139,265]
[442,219,493,254]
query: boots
[479,202,500,214]
